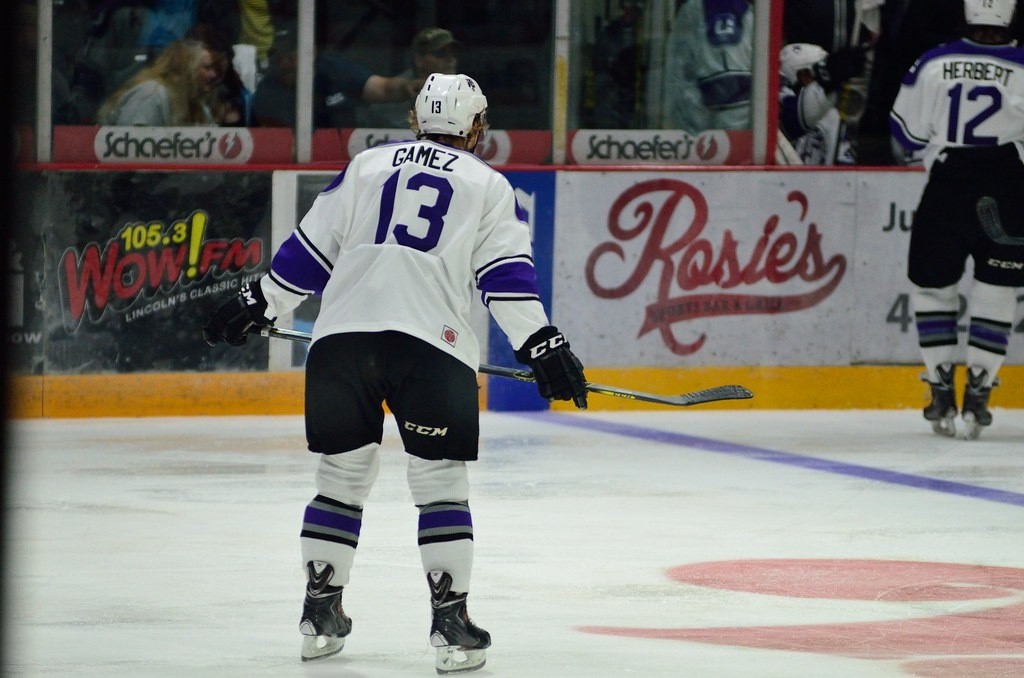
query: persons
[886,0,1024,440]
[204,74,586,675]
[22,0,965,376]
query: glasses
[432,46,454,57]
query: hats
[411,27,457,53]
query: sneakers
[919,363,960,437]
[426,570,491,674]
[299,562,353,662]
[962,367,1003,439]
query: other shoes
[174,349,214,371]
[115,329,149,372]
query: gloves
[201,280,277,347]
[811,47,868,94]
[514,326,588,410]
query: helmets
[780,43,829,85]
[965,0,1018,28]
[414,72,489,136]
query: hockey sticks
[975,195,1024,245]
[249,324,755,408]
